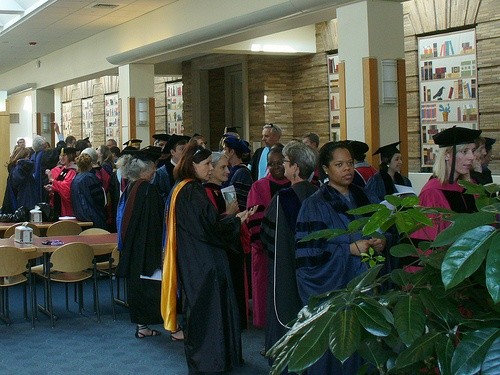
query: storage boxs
[30,210,43,223]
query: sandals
[171,328,185,342]
[138,326,161,337]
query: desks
[0,222,94,236]
[0,234,119,325]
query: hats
[344,140,369,160]
[163,134,190,153]
[223,127,238,134]
[152,133,171,141]
[123,139,142,146]
[372,141,401,161]
[432,125,482,148]
[81,148,98,163]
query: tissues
[30,205,43,223]
[14,222,34,243]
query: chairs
[0,220,120,329]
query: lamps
[42,113,49,132]
[380,58,397,105]
[6,82,36,97]
[107,0,346,64]
[137,98,147,126]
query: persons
[0,139,143,234]
[118,125,421,375]
[404,126,500,291]
